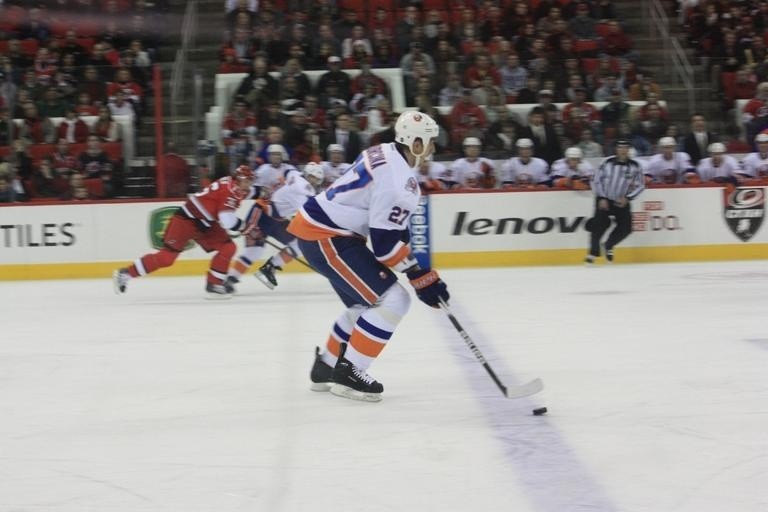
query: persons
[551,146,596,188]
[286,112,451,402]
[741,134,768,182]
[584,139,645,264]
[627,147,648,187]
[500,138,550,191]
[111,164,257,296]
[420,154,447,191]
[317,144,353,194]
[223,162,325,295]
[0,0,171,201]
[698,143,740,183]
[447,135,497,190]
[251,145,297,199]
[644,137,700,185]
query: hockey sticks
[437,298,543,399]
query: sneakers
[310,346,334,384]
[603,242,613,262]
[334,342,383,393]
[227,275,239,284]
[584,254,596,263]
[117,267,128,295]
[206,283,234,294]
[259,256,283,286]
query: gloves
[245,223,264,241]
[407,269,449,309]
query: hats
[230,96,251,105]
[534,90,553,98]
[529,107,545,114]
[282,99,302,109]
[573,84,587,91]
[327,56,341,62]
[610,87,622,96]
[252,51,269,58]
[647,91,658,99]
[545,104,560,112]
[331,98,347,105]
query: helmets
[754,134,768,142]
[706,143,727,153]
[462,137,481,145]
[657,136,678,146]
[515,139,534,148]
[234,165,256,179]
[267,144,290,161]
[612,138,632,147]
[302,162,324,184]
[326,144,346,153]
[565,147,583,157]
[395,110,440,157]
[627,148,637,156]
